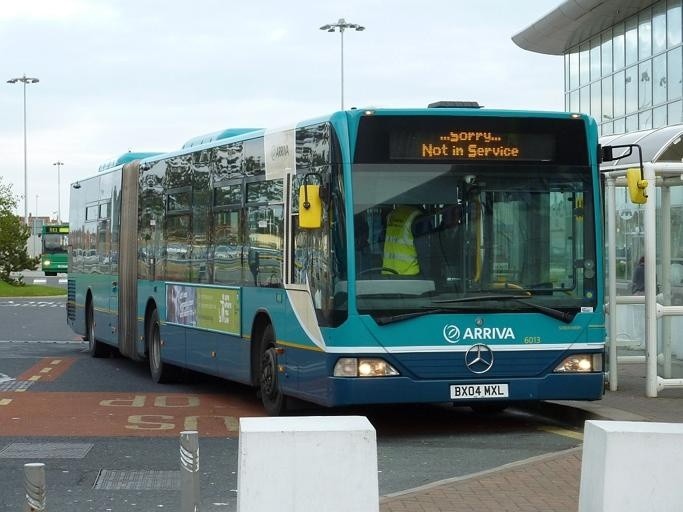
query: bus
[68,99,648,416]
[37,223,70,277]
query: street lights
[317,17,367,115]
[51,161,65,224]
[7,73,40,247]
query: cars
[72,244,265,266]
[548,239,636,268]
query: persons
[376,204,425,275]
[431,206,475,293]
[632,257,659,296]
